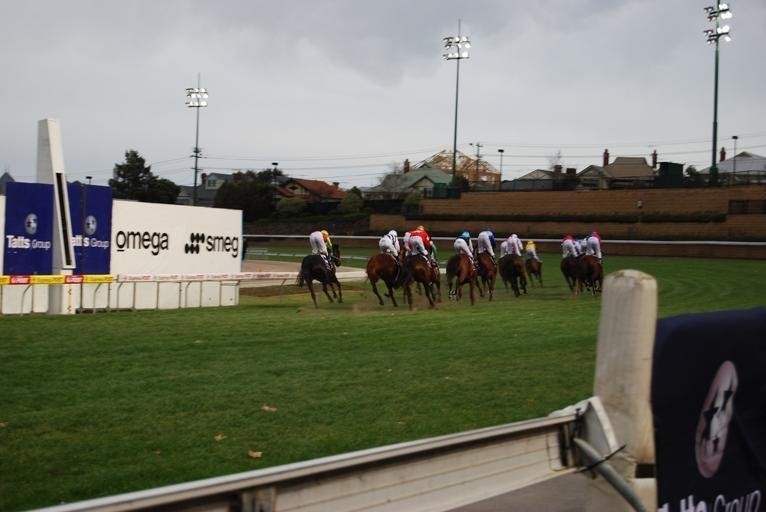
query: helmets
[322,230,328,237]
[417,226,424,230]
[528,240,533,244]
[487,228,491,231]
[462,231,470,238]
[511,233,518,238]
[389,230,398,237]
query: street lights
[443,18,471,182]
[183,85,209,203]
[85,175,92,184]
[731,134,738,175]
[703,0,734,174]
[498,150,505,181]
[271,161,278,198]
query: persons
[377,229,401,265]
[309,229,334,264]
[403,225,603,266]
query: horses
[561,254,604,297]
[498,253,527,298]
[445,243,497,306]
[296,243,343,309]
[525,247,546,288]
[366,239,422,307]
[399,237,441,311]
